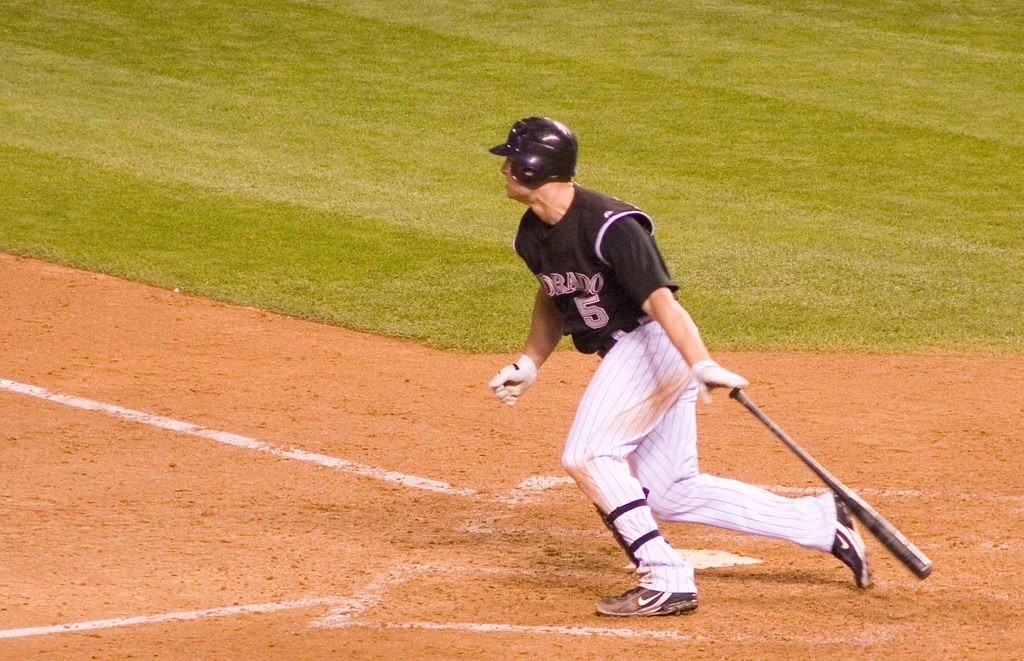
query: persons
[487,118,872,618]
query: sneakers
[831,491,869,588]
[596,584,699,615]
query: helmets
[489,116,577,187]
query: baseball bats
[727,385,935,582]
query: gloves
[490,354,538,405]
[692,360,749,404]
